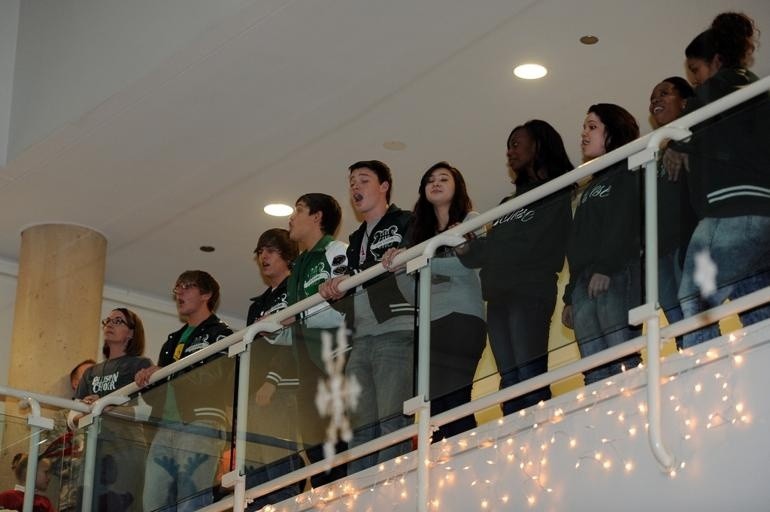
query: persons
[0,452,57,512]
[662,10,770,348]
[42,356,96,512]
[63,306,154,512]
[645,71,709,347]
[316,159,418,472]
[560,101,647,384]
[258,193,350,490]
[132,268,240,512]
[380,161,487,442]
[243,222,307,512]
[446,119,579,415]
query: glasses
[101,316,131,328]
[169,282,204,295]
